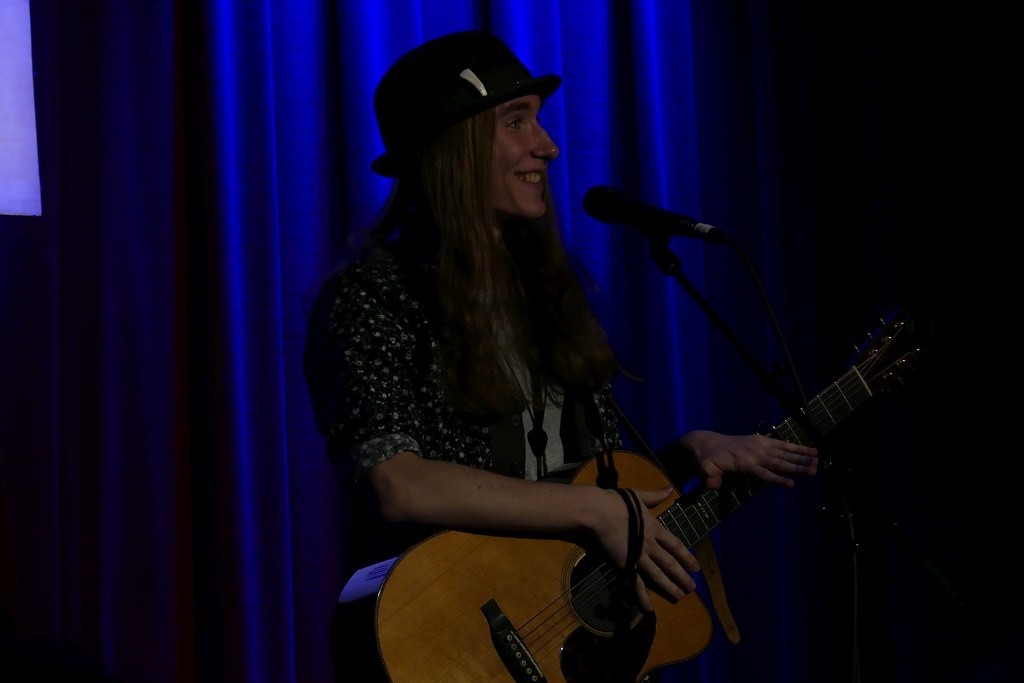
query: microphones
[583,185,723,244]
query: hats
[371,29,564,179]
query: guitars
[334,302,931,682]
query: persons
[301,33,821,617]
